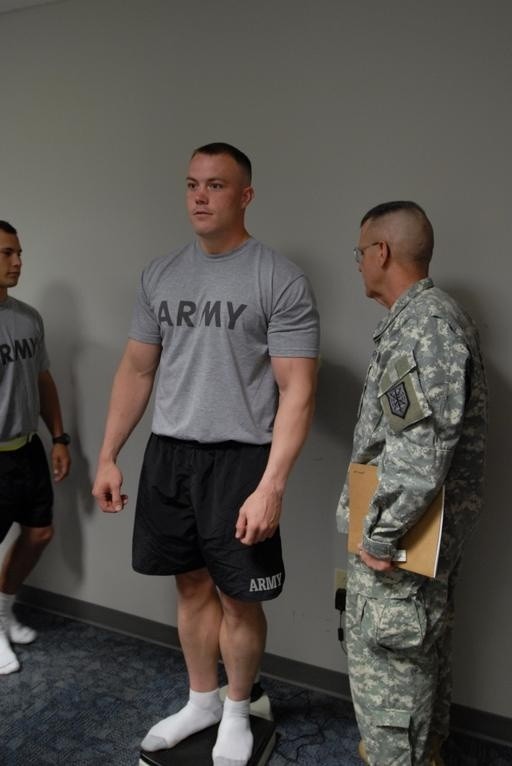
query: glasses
[352,242,382,262]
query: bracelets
[52,433,71,446]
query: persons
[0,218,74,677]
[327,200,486,766]
[90,142,319,765]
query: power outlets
[336,571,346,610]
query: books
[348,459,450,580]
